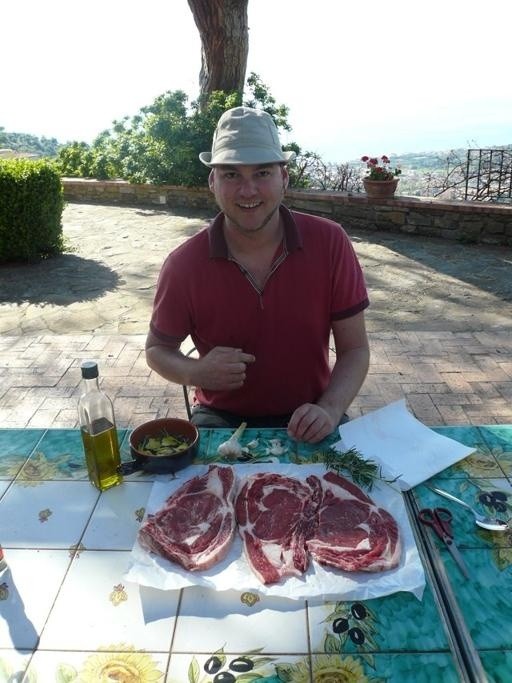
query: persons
[145,104,371,446]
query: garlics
[217,441,241,459]
[266,439,288,456]
[268,457,280,464]
[246,441,258,450]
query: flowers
[361,154,403,179]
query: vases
[363,177,399,197]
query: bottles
[77,361,124,491]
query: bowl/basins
[128,417,199,473]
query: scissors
[418,508,469,581]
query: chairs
[178,346,200,429]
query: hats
[199,107,297,167]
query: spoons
[425,481,510,531]
